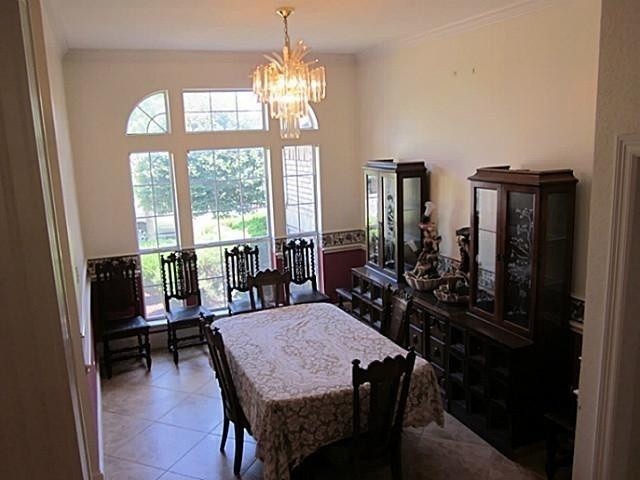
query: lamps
[252,8,325,138]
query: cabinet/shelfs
[335,158,584,480]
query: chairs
[161,251,215,367]
[200,319,253,477]
[94,257,152,379]
[379,287,413,344]
[225,246,274,316]
[326,352,414,480]
[282,239,331,305]
[247,269,291,312]
